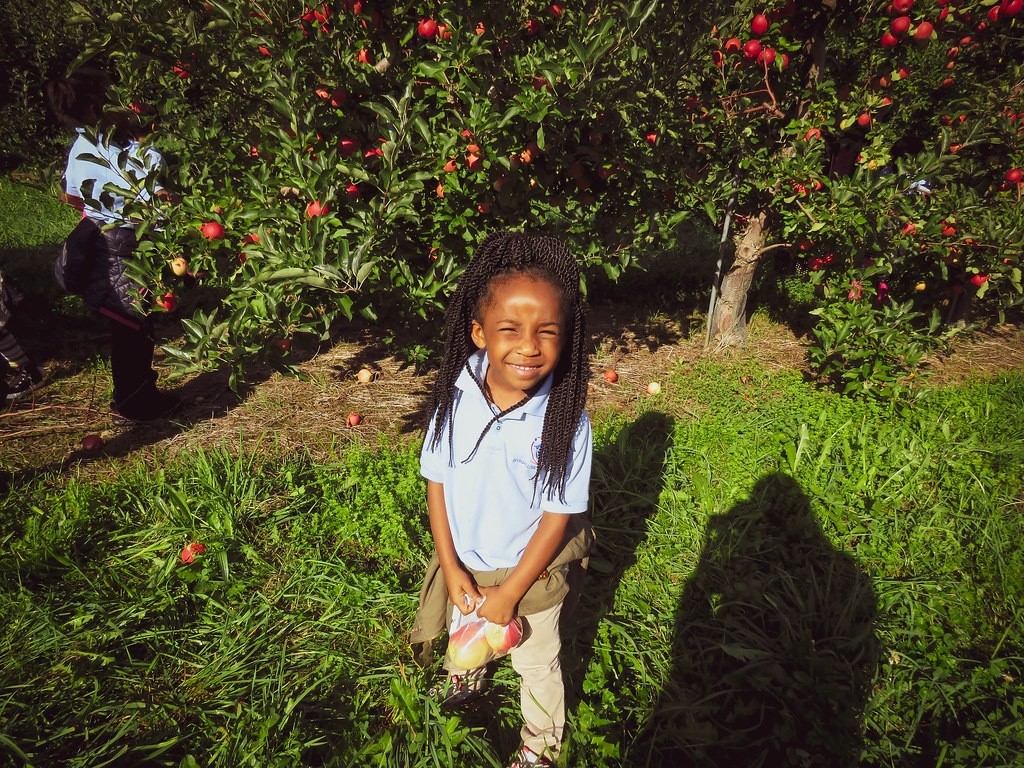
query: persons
[0,268,47,400]
[408,229,595,768]
[47,66,185,427]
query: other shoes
[114,392,178,420]
[5,366,45,399]
[429,672,488,707]
[509,746,551,768]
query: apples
[485,616,523,652]
[182,543,206,564]
[447,622,492,670]
[83,435,102,452]
[118,0,1024,426]
